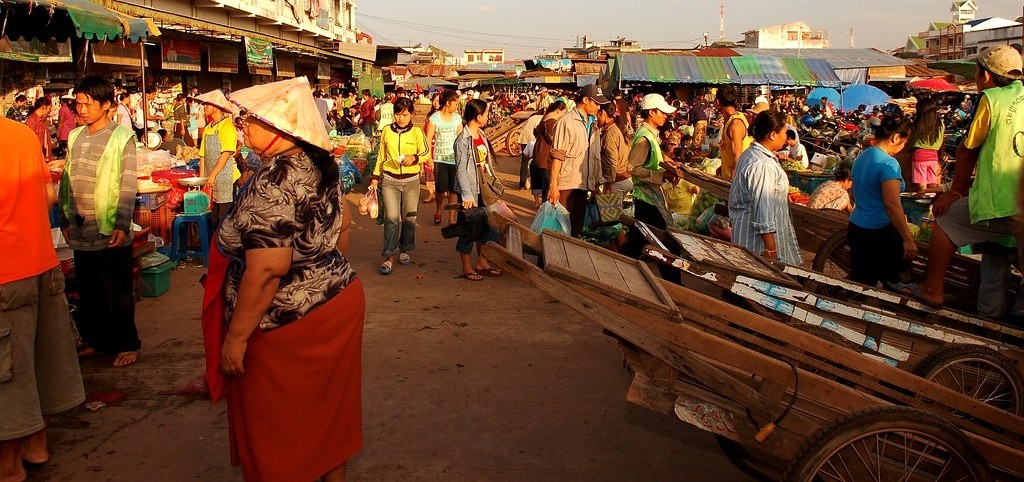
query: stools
[170,212,213,267]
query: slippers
[379,261,393,274]
[399,253,410,265]
[434,214,441,224]
[112,349,142,368]
[459,271,483,281]
[475,266,502,277]
[886,280,943,307]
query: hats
[192,89,233,114]
[226,75,334,152]
[61,88,76,99]
[579,83,611,105]
[977,45,1024,80]
[638,93,677,114]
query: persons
[455,99,503,281]
[60,75,142,367]
[911,98,945,190]
[847,111,918,285]
[370,97,431,274]
[547,84,611,238]
[0,81,975,275]
[886,43,1024,309]
[0,113,87,482]
[200,75,367,482]
[728,109,805,267]
[618,93,686,287]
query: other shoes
[423,194,435,203]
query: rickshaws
[481,209,1024,482]
[485,108,537,157]
[669,159,1024,324]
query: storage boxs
[55,167,214,298]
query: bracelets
[764,249,777,255]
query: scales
[178,175,211,216]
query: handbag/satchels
[356,185,384,225]
[530,201,572,236]
[474,144,504,206]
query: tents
[0,0,163,147]
